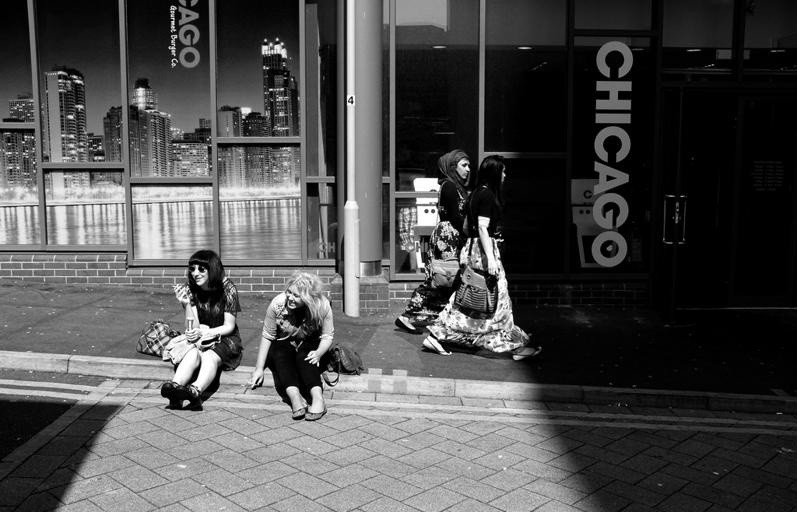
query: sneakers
[292,402,307,419]
[305,405,327,421]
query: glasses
[188,266,204,272]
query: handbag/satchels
[330,342,364,375]
[428,257,460,289]
[454,267,497,313]
[136,320,179,357]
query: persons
[388,147,471,336]
[420,155,543,363]
[160,247,244,414]
[239,268,337,424]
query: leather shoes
[161,383,182,409]
[176,385,203,411]
[513,347,542,361]
[422,335,453,356]
[395,318,423,334]
